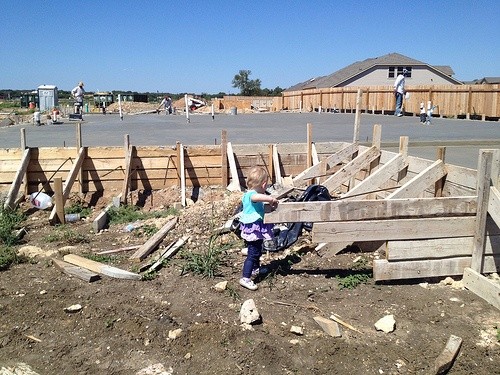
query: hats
[402,69,408,74]
[78,82,84,86]
[164,96,169,100]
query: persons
[420,103,426,125]
[392,69,408,117]
[155,96,172,114]
[238,166,279,291]
[71,82,85,115]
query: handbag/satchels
[405,92,410,100]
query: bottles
[30,192,52,209]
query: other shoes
[239,278,258,290]
[396,113,402,117]
[252,268,268,276]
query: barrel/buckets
[231,107,237,115]
